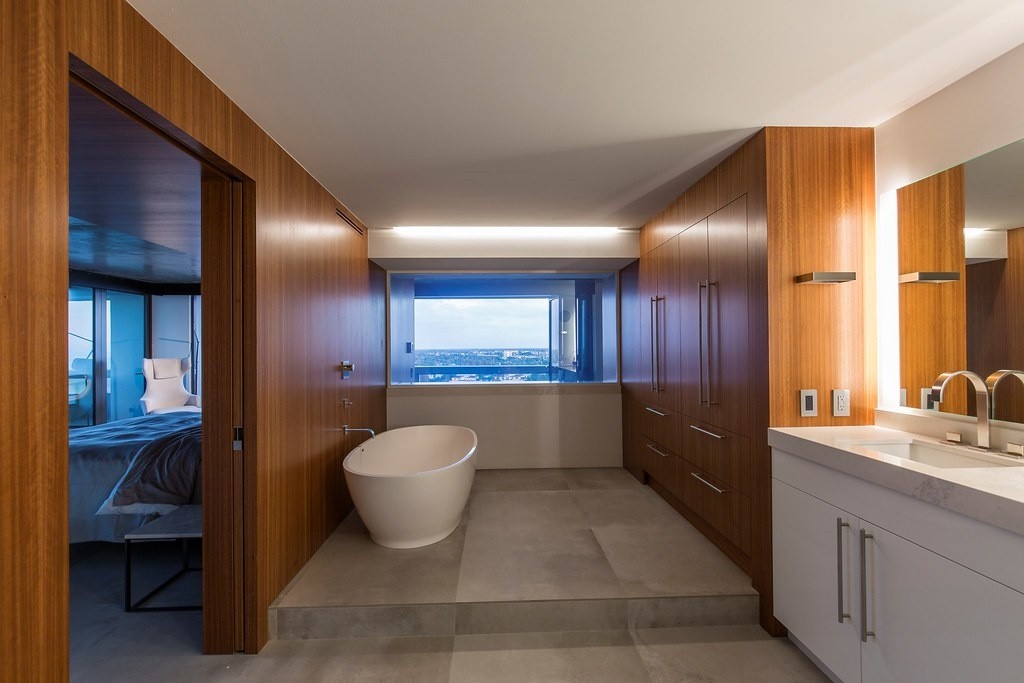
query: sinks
[839,438,1024,469]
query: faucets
[984,369,1024,420]
[343,425,376,439]
[931,370,993,452]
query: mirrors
[896,138,1024,425]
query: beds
[66,411,211,546]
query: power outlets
[900,389,907,407]
[832,389,851,416]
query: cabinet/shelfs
[637,235,679,412]
[772,476,1024,683]
[635,401,679,499]
[678,413,751,557]
[679,192,748,439]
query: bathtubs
[342,424,480,549]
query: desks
[122,504,204,614]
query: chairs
[140,355,203,418]
